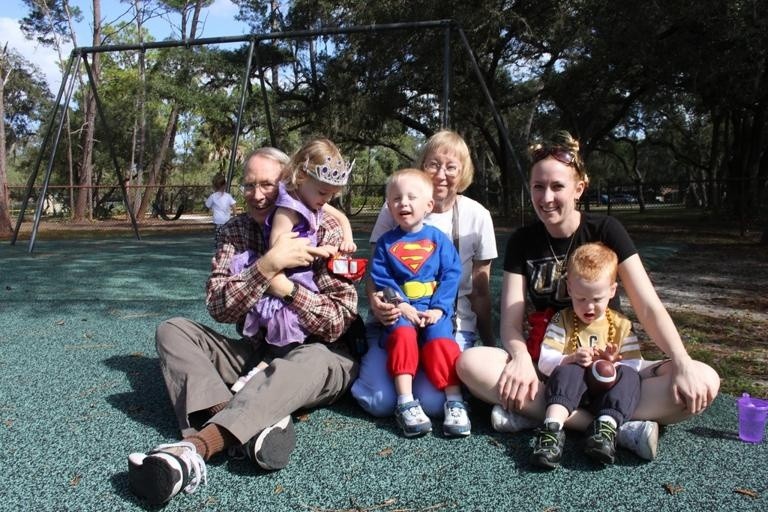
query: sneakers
[238,413,297,471]
[489,403,544,434]
[617,420,660,461]
[392,397,433,439]
[127,440,203,506]
[580,418,618,465]
[525,421,566,470]
[441,400,472,436]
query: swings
[140,40,191,222]
[324,25,378,216]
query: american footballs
[586,359,618,392]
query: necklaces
[545,227,577,273]
[568,308,615,356]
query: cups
[738,392,768,443]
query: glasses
[528,147,582,175]
[423,160,463,178]
[238,183,279,195]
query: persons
[455,130,721,461]
[227,139,357,393]
[529,243,642,470]
[368,168,471,438]
[351,130,498,418]
[204,172,236,248]
[127,146,368,506]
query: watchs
[282,280,298,306]
